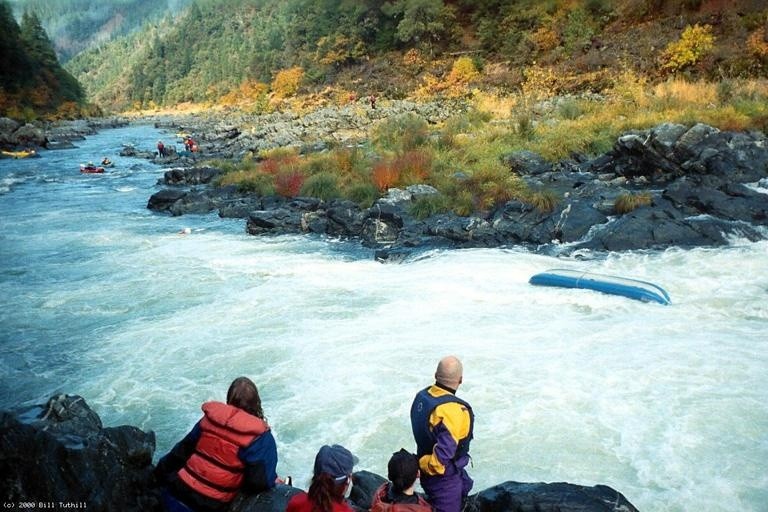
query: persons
[79,157,111,172]
[184,136,200,155]
[285,358,474,512]
[158,140,164,158]
[155,377,278,512]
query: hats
[313,444,359,482]
[388,447,419,481]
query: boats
[80,159,116,173]
[1,149,35,158]
[528,267,673,305]
